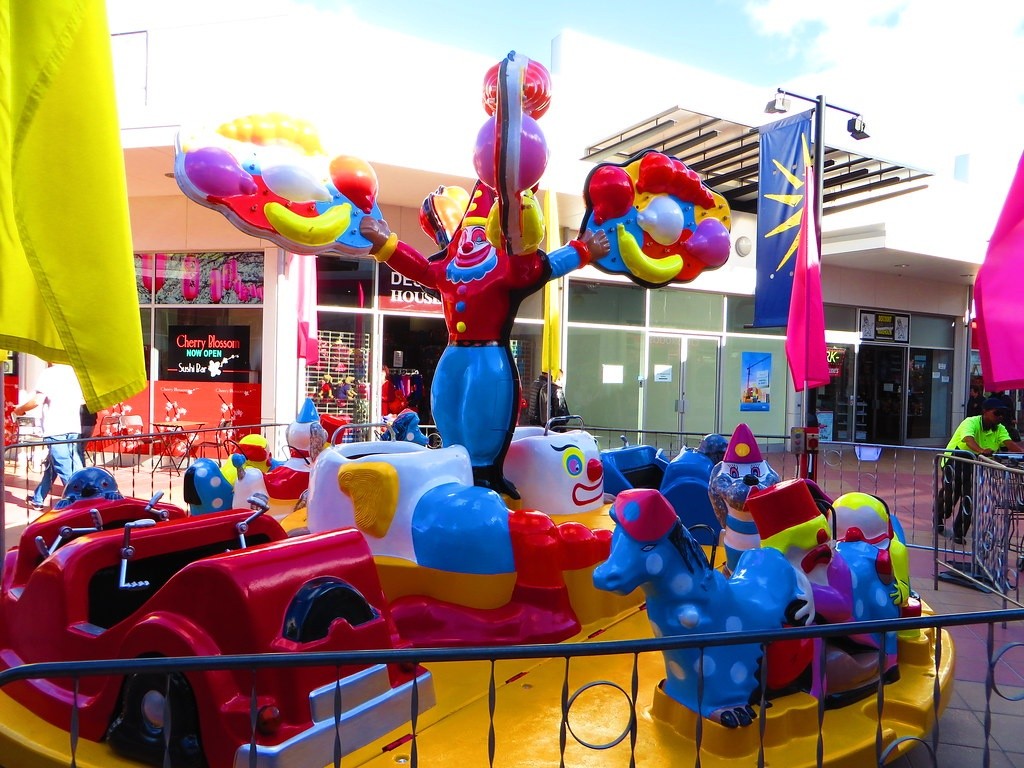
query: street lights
[763,88,870,480]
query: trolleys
[980,451,1024,571]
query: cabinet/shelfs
[835,390,867,440]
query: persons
[966,387,1018,452]
[13,362,98,512]
[938,398,1024,544]
[360,188,611,489]
[528,370,570,433]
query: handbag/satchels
[1008,422,1021,443]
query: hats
[982,398,1008,410]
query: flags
[973,152,1024,392]
[785,166,830,393]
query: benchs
[96,528,374,627]
[621,464,664,491]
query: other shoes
[933,517,944,534]
[25,496,44,511]
[953,536,966,545]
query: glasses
[988,411,1004,416]
